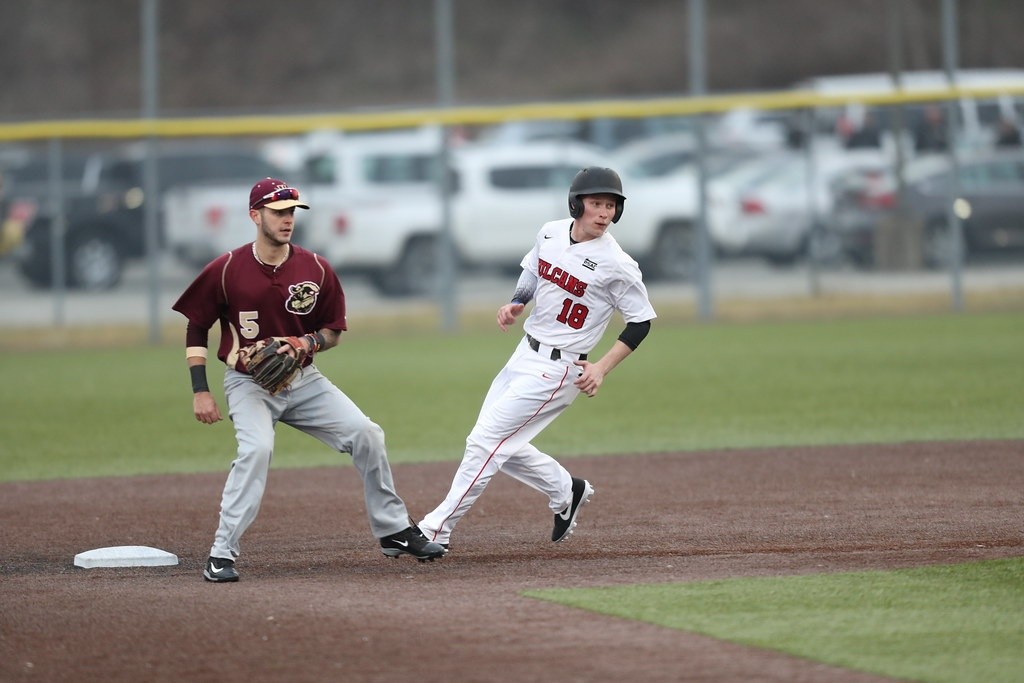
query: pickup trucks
[164,130,717,298]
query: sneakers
[411,525,448,553]
[551,477,595,543]
[203,556,240,583]
[380,527,447,565]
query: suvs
[15,149,291,295]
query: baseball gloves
[236,336,304,397]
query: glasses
[251,188,298,205]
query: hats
[248,176,309,211]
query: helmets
[568,165,626,223]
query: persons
[171,175,446,584]
[412,165,656,550]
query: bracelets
[304,333,320,354]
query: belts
[526,333,587,362]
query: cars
[624,68,1023,266]
